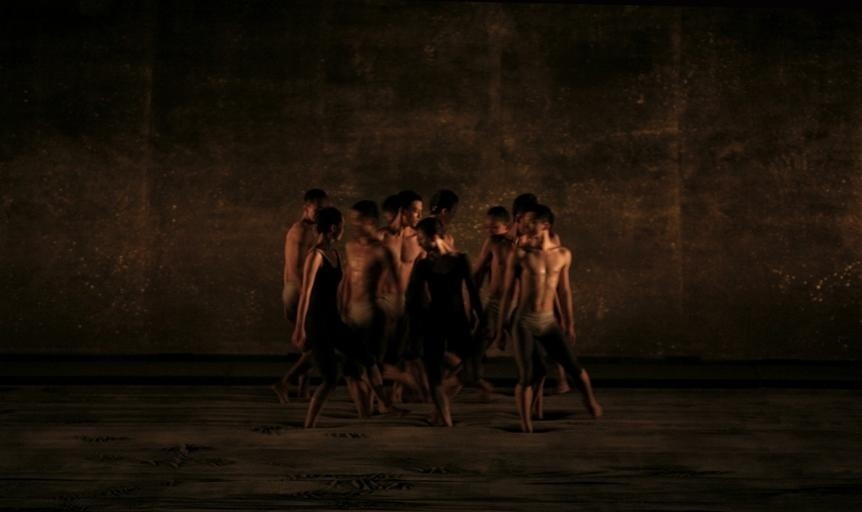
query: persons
[271,187,605,435]
